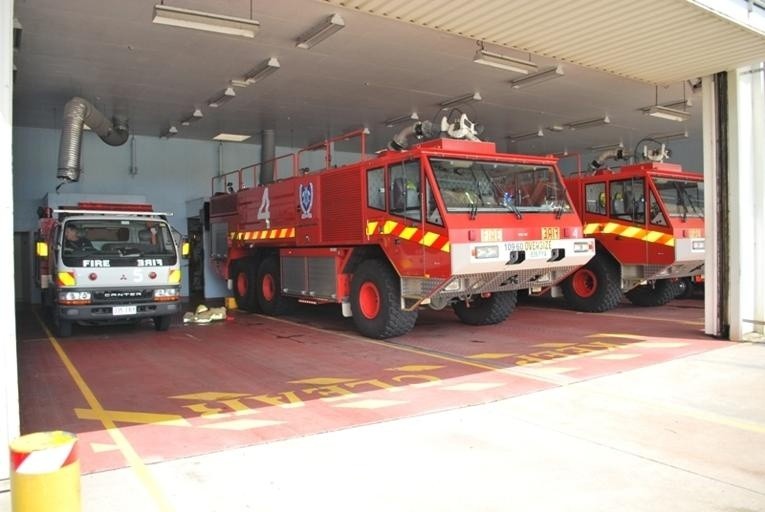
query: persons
[61,221,95,255]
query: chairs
[394,177,420,212]
[104,229,157,253]
[600,188,646,222]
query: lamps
[151,1,259,40]
[649,80,691,122]
[474,41,539,76]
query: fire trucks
[33,190,184,331]
[190,112,598,342]
[554,161,708,313]
